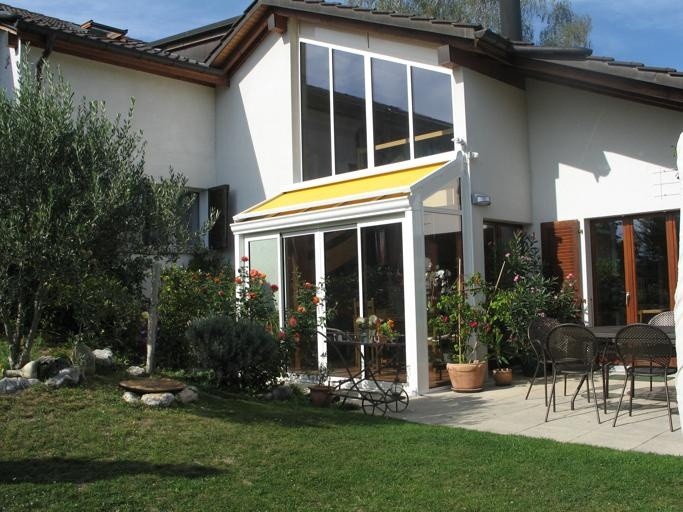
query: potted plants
[308,361,335,407]
[480,328,513,387]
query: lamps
[470,192,491,208]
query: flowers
[435,229,580,360]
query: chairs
[353,298,402,375]
[524,311,678,432]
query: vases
[446,362,486,392]
